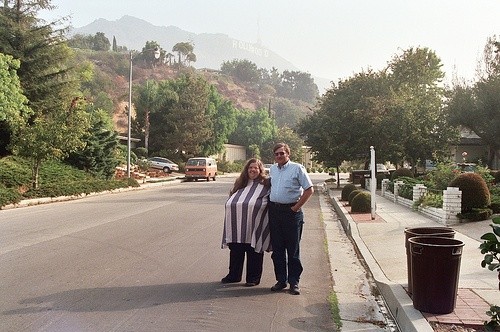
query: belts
[270,202,296,207]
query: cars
[142,156,179,173]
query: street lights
[126,49,160,175]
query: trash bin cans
[403,226,455,295]
[408,236,464,315]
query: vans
[185,158,221,180]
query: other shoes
[246,282,256,286]
[289,284,300,295]
[271,281,287,291]
[221,277,240,283]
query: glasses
[273,152,286,156]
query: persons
[267,144,314,295]
[222,158,271,285]
